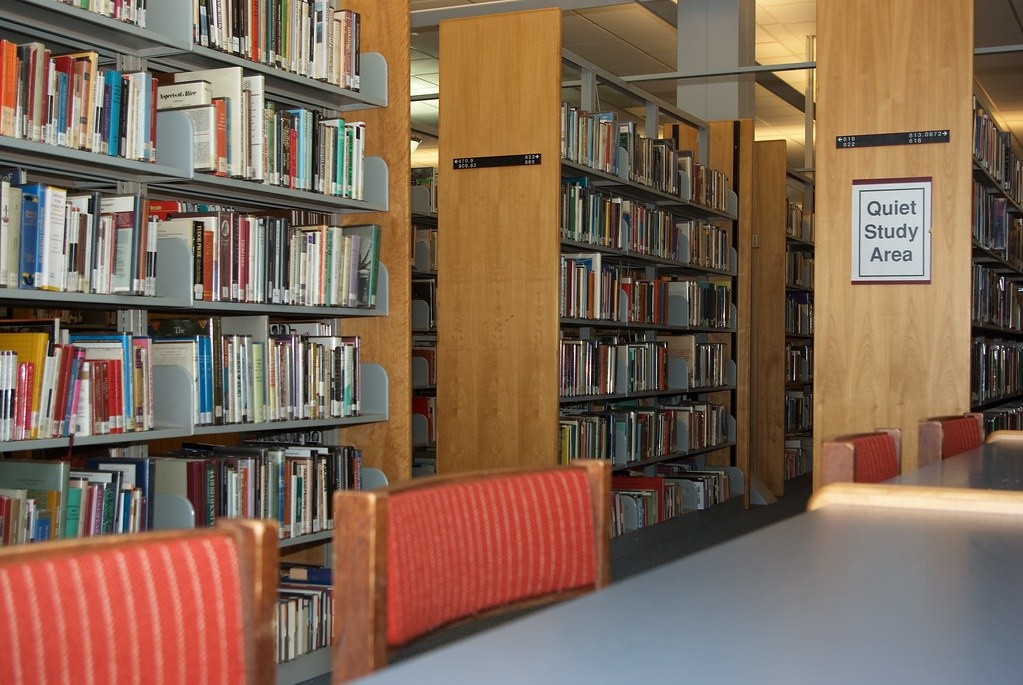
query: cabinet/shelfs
[0,0,1023,685]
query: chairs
[0,518,280,685]
[917,410,986,468]
[331,459,611,685]
[820,428,904,484]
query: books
[560,174,730,271]
[0,443,363,540]
[783,197,815,482]
[560,100,729,213]
[273,560,335,664]
[0,317,361,441]
[559,248,733,330]
[0,39,366,200]
[559,400,730,542]
[57,1,362,92]
[559,333,725,396]
[971,97,1023,435]
[0,163,382,309]
[410,163,438,479]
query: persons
[311,431,319,442]
[305,432,310,441]
[293,432,300,442]
[285,433,291,442]
[279,434,285,442]
[272,435,278,442]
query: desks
[338,491,1023,685]
[877,438,1023,492]
